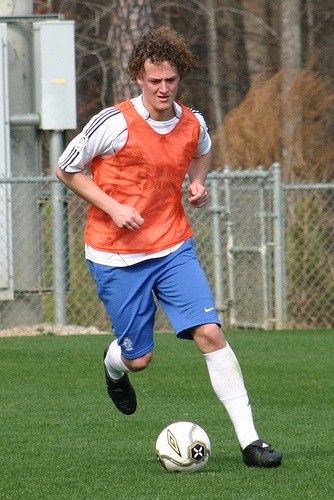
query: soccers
[155,420,211,474]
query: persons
[55,26,284,468]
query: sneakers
[103,347,137,415]
[239,439,282,468]
